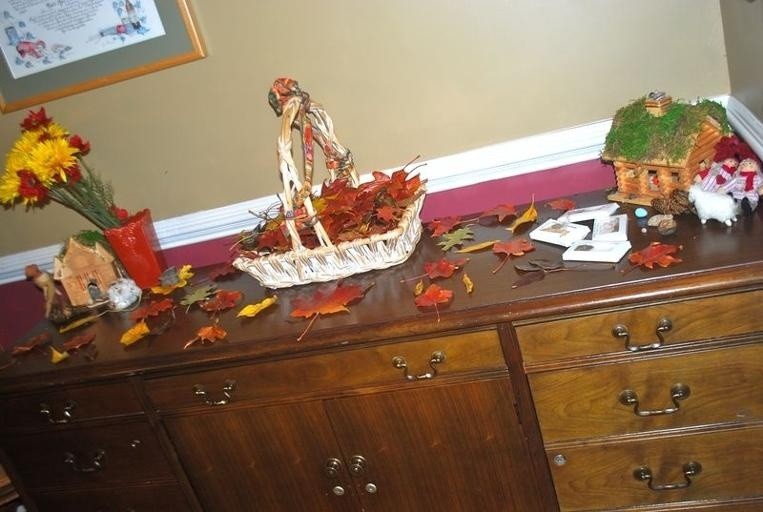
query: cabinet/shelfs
[0,187,762,512]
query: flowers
[0,107,125,230]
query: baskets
[233,98,428,290]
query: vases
[104,209,168,289]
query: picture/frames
[0,0,208,113]
